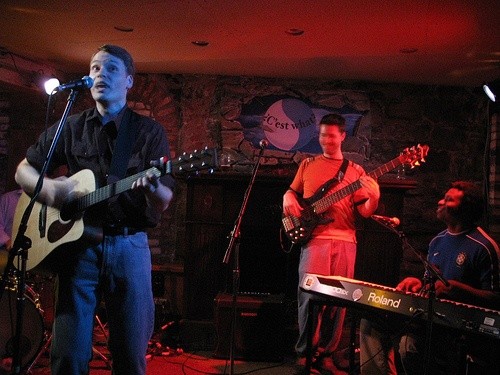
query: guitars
[281,143,429,244]
[10,147,219,272]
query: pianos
[299,272,500,375]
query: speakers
[211,293,286,363]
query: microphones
[373,214,400,227]
[259,139,268,148]
[54,76,94,89]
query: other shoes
[295,358,348,375]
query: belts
[104,224,145,236]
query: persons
[282,113,380,374]
[15,44,174,375]
[0,172,47,251]
[360,181,500,375]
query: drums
[0,274,53,372]
